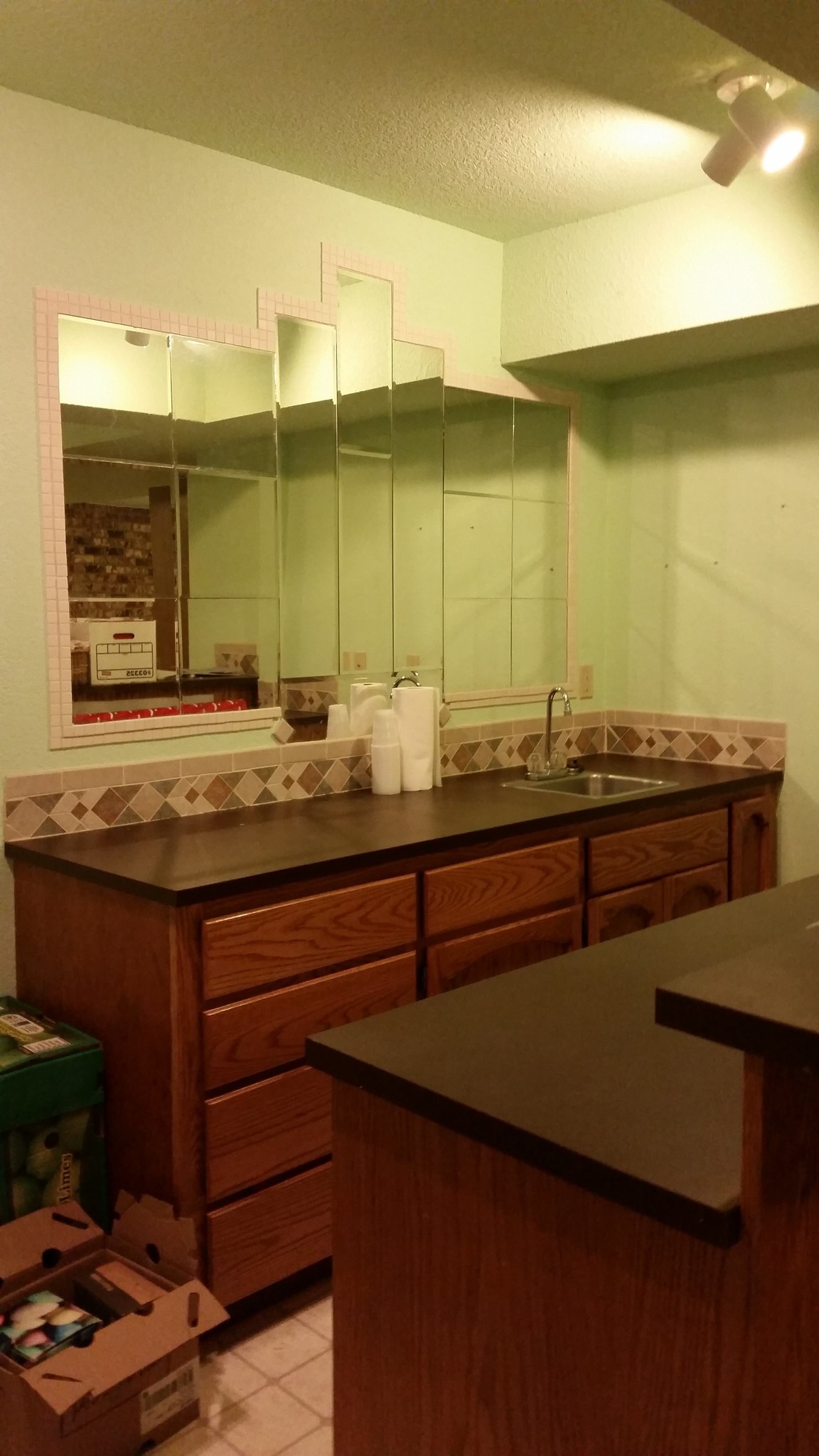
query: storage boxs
[70,617,156,686]
[70,640,91,686]
[0,1190,230,1456]
[0,994,114,1236]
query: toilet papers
[393,686,443,792]
[350,682,387,736]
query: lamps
[701,64,807,188]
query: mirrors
[33,243,578,749]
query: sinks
[500,770,679,799]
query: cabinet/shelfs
[15,782,819,1456]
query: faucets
[525,685,573,781]
